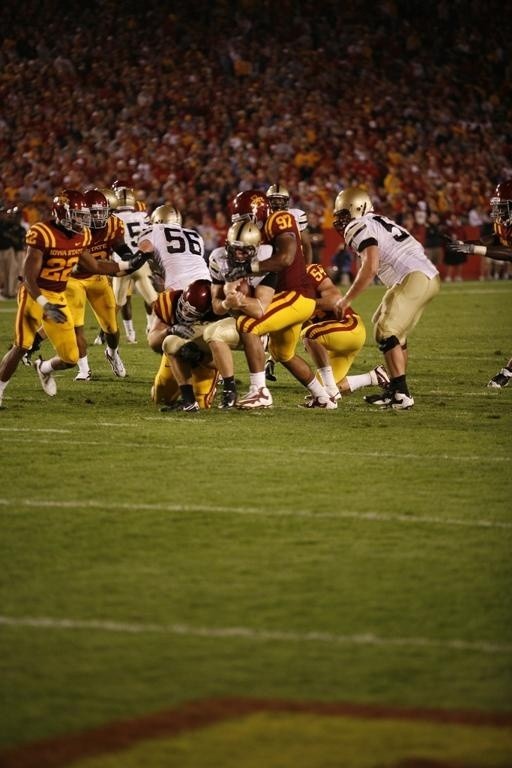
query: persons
[1,0,511,298]
[1,178,511,412]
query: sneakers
[12,327,137,397]
[158,359,415,414]
[487,366,512,389]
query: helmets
[489,181,512,228]
[175,282,212,325]
[51,180,182,236]
[225,184,375,268]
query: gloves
[223,266,247,282]
[447,243,474,256]
[116,244,165,292]
[44,303,67,324]
[171,324,195,340]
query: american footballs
[237,281,251,296]
